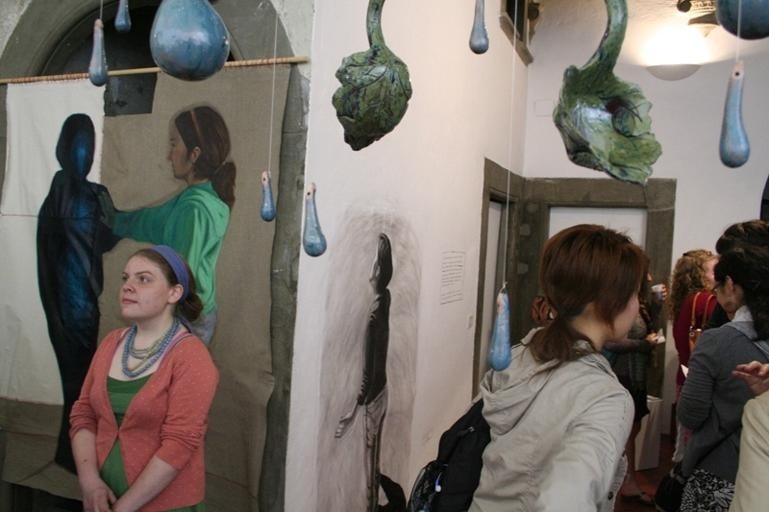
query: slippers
[621,491,654,507]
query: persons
[68,244,220,511]
[333,230,407,511]
[35,112,121,474]
[97,104,236,346]
[463,221,768,512]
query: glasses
[711,281,724,297]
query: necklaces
[121,316,180,378]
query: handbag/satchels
[404,399,490,511]
[689,328,703,351]
[655,475,684,512]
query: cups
[652,285,667,305]
